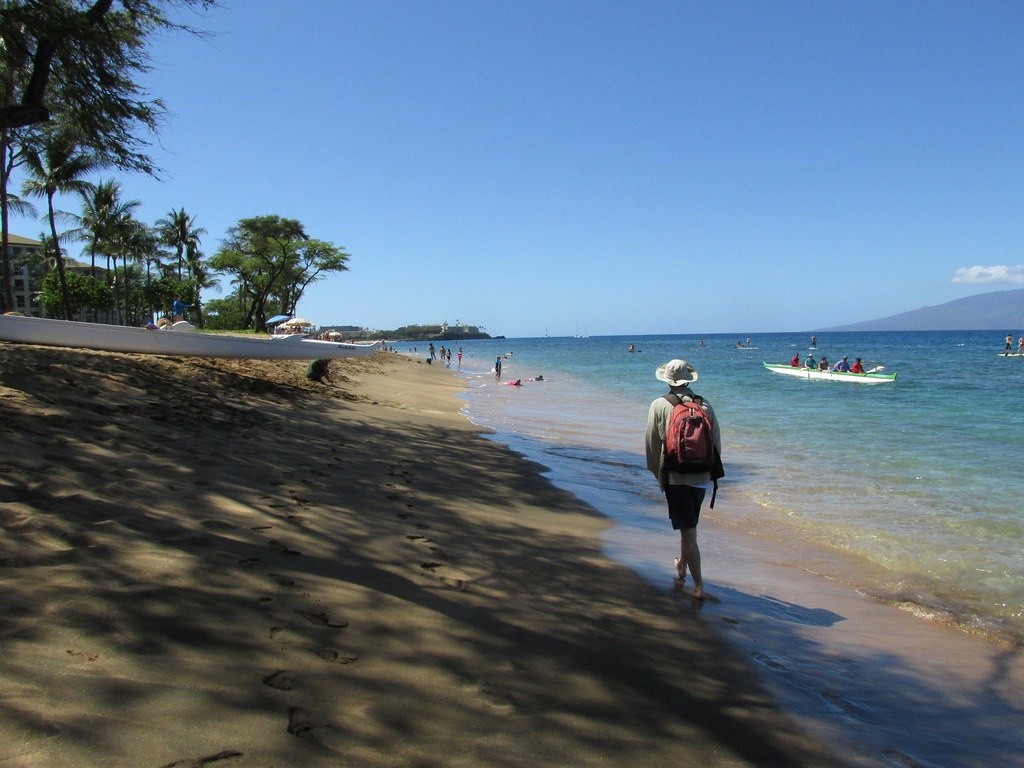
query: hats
[808,354,813,357]
[655,359,698,386]
[843,356,847,360]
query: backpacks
[662,393,716,468]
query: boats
[762,360,898,383]
[997,353,1024,357]
[738,348,759,350]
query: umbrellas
[330,331,343,337]
[266,316,313,335]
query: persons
[834,355,851,372]
[804,353,818,369]
[747,337,751,347]
[524,375,544,382]
[429,342,437,361]
[446,348,453,363]
[818,356,830,371]
[274,324,304,336]
[1018,335,1024,354]
[851,357,867,373]
[1005,333,1012,353]
[495,357,502,379]
[318,329,355,345]
[381,341,417,353]
[306,359,332,385]
[645,358,722,599]
[173,293,195,323]
[457,347,463,366]
[811,335,816,348]
[495,378,523,386]
[790,352,801,368]
[440,345,446,360]
